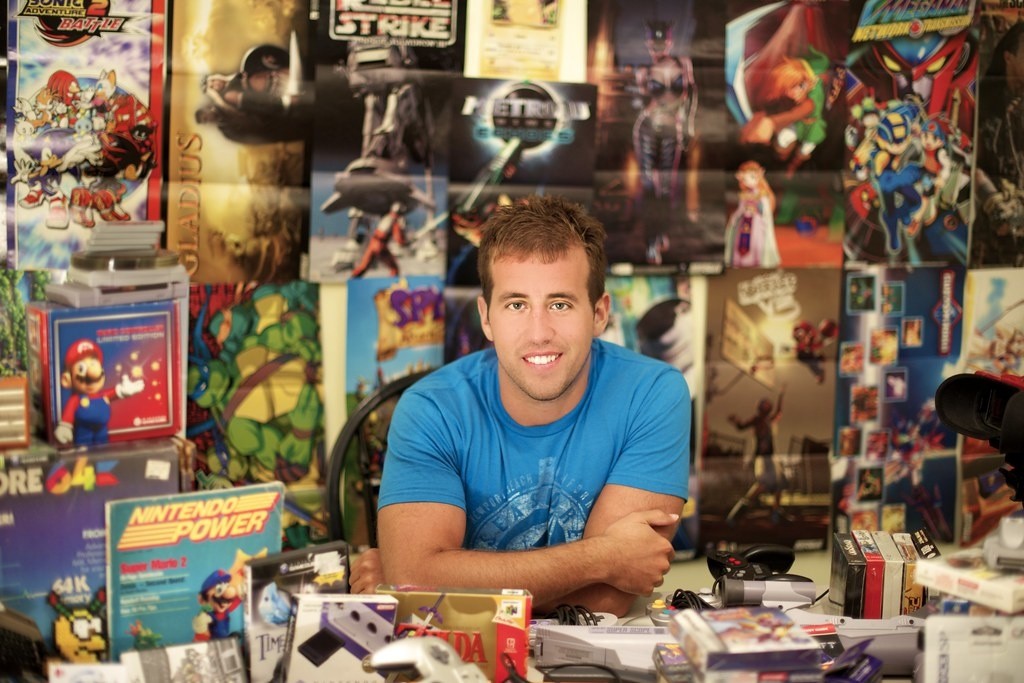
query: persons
[349,193,691,618]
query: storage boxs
[24,301,184,450]
[279,593,399,683]
[375,582,533,683]
[0,434,180,660]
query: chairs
[325,370,431,548]
